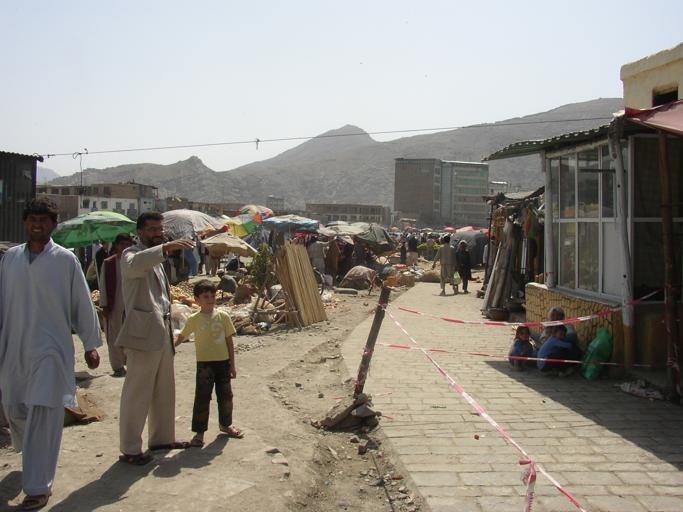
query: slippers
[149,439,190,450]
[191,436,204,447]
[119,453,151,465]
[219,424,244,437]
[22,491,51,510]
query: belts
[163,312,171,320]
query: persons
[455,240,472,294]
[432,236,459,295]
[0,198,103,510]
[174,280,244,448]
[508,326,533,371]
[538,306,582,364]
[483,236,497,284]
[399,232,440,263]
[115,213,196,466]
[100,233,135,377]
[537,325,575,378]
